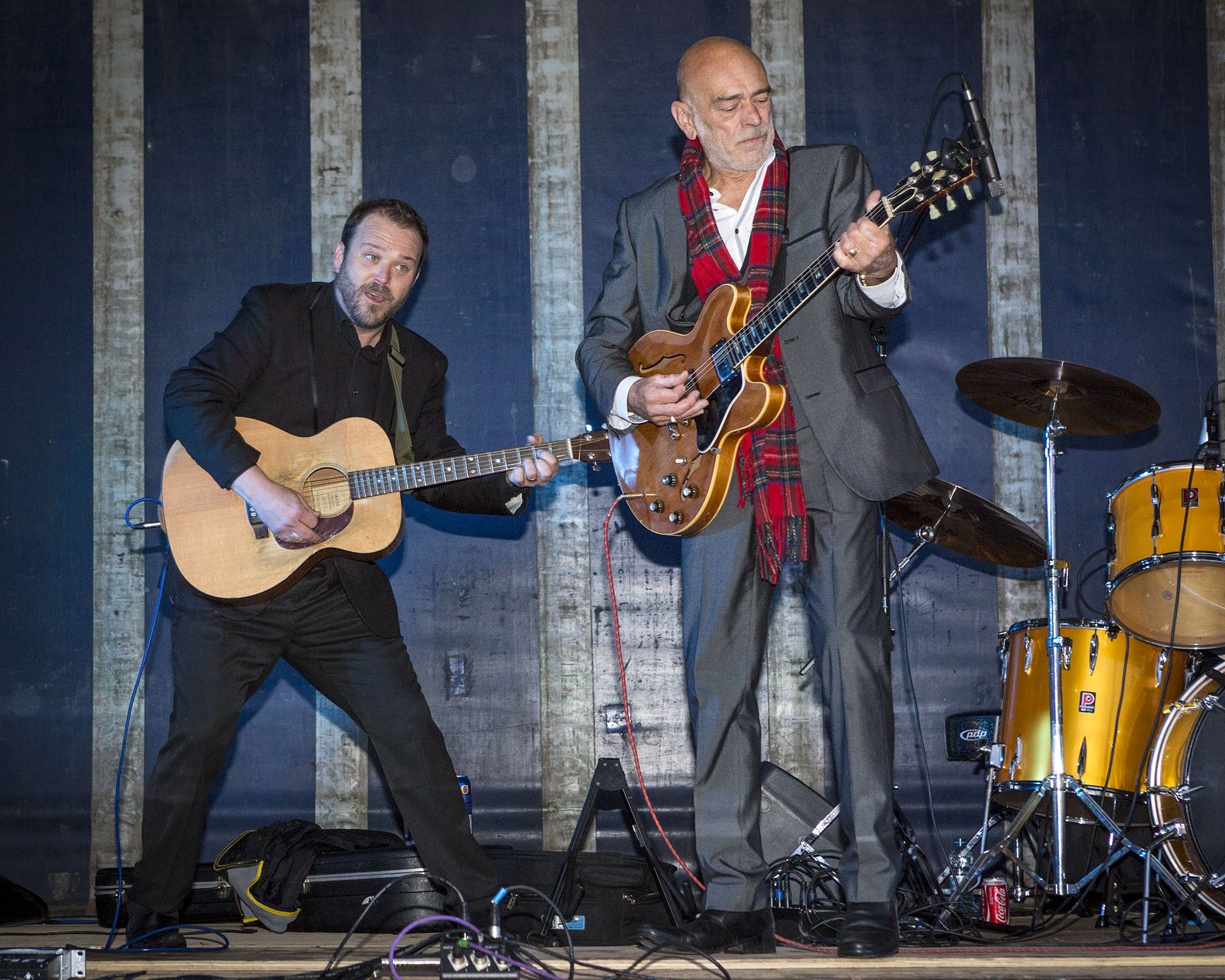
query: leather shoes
[125,915,187,949]
[837,897,900,959]
[637,908,776,955]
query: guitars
[160,417,611,606]
[612,142,977,538]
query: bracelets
[858,272,868,287]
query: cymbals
[954,357,1161,436]
[881,479,1048,567]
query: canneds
[981,877,1010,925]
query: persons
[568,33,941,957]
[121,199,563,960]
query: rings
[669,416,677,423]
[526,475,538,481]
[289,531,299,540]
[845,245,861,260]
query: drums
[1149,661,1225,915]
[1107,459,1225,651]
[992,617,1188,827]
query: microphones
[960,78,1008,200]
[1198,388,1216,462]
